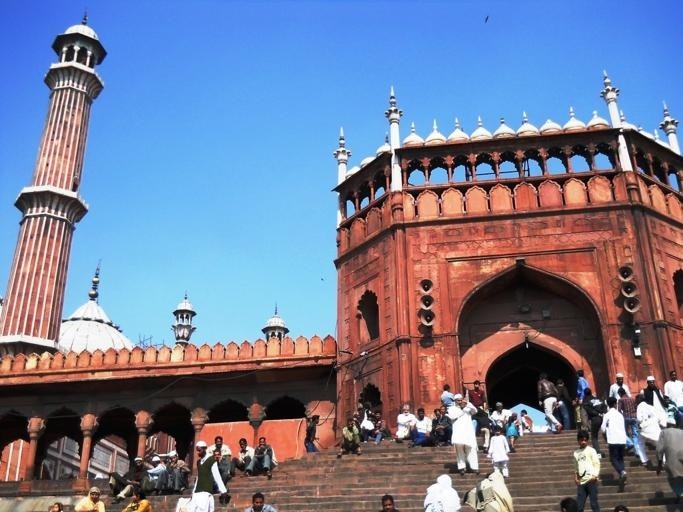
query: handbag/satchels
[360,419,374,430]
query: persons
[302,380,535,475]
[377,468,528,512]
[535,366,682,512]
[50,427,279,512]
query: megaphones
[418,278,435,329]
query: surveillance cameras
[359,351,369,357]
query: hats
[647,376,655,381]
[453,394,463,400]
[615,373,624,379]
[195,441,207,447]
[135,451,176,462]
